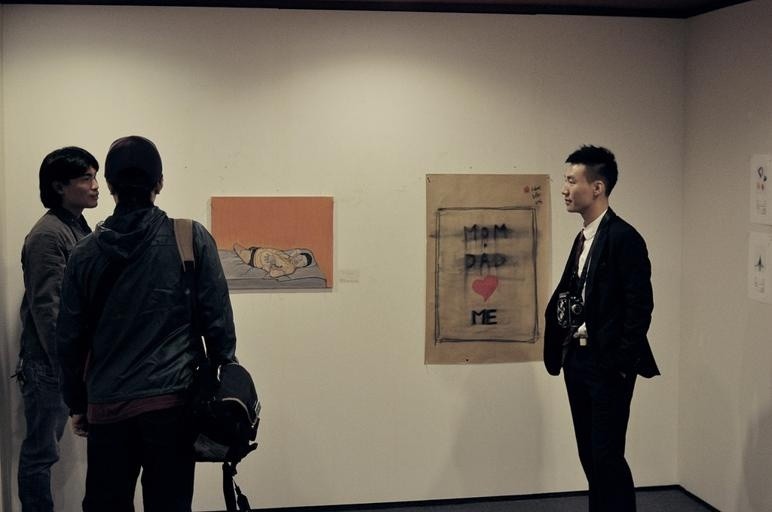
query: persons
[57,136,239,512]
[544,145,661,512]
[17,146,99,512]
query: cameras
[556,291,582,328]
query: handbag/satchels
[172,216,262,509]
